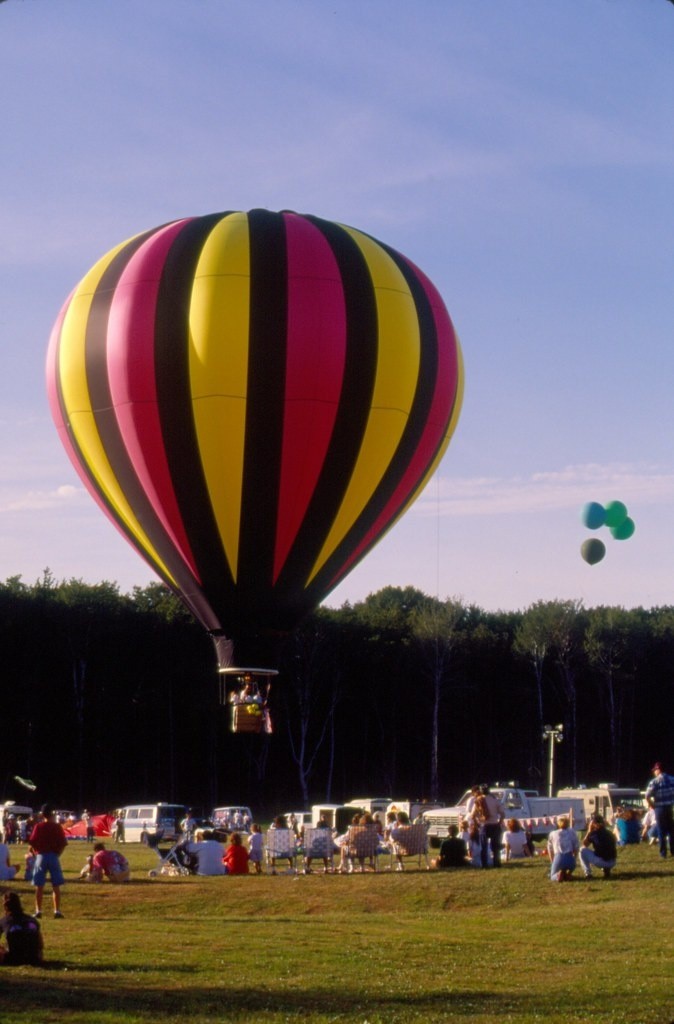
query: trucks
[418,784,589,855]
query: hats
[8,814,15,819]
[593,816,604,823]
[41,804,56,813]
[650,762,664,773]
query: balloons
[581,539,605,565]
[582,500,635,541]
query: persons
[79,843,130,883]
[68,809,94,843]
[548,817,581,883]
[431,784,504,868]
[502,818,535,862]
[0,809,44,882]
[28,803,70,920]
[266,813,384,874]
[230,684,262,704]
[114,812,126,844]
[180,807,250,877]
[580,814,616,879]
[0,891,46,968]
[614,763,674,860]
[385,812,425,870]
[248,824,264,873]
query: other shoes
[34,912,41,918]
[585,874,592,879]
[396,866,404,872]
[603,870,610,877]
[54,913,64,918]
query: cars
[210,806,254,834]
[266,811,312,839]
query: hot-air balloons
[41,205,467,736]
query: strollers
[140,828,191,877]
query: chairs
[387,823,431,871]
[302,827,335,874]
[265,827,297,875]
[344,823,380,874]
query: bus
[109,801,187,845]
